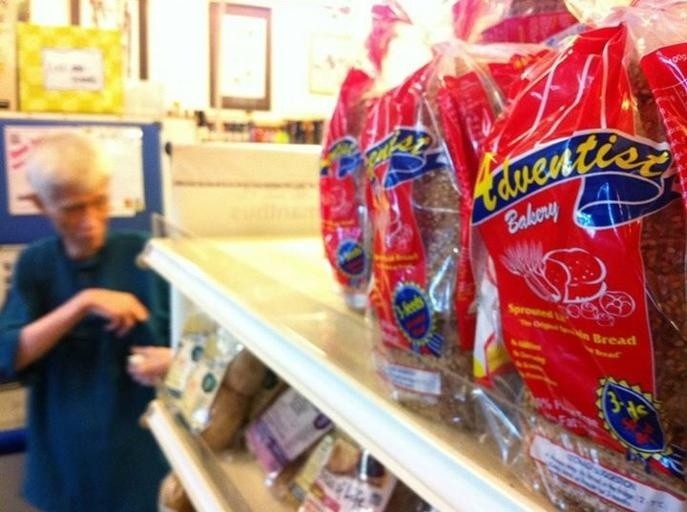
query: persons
[0,131,171,512]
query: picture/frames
[68,0,150,82]
[206,0,273,111]
[15,23,126,118]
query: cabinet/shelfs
[133,235,593,512]
